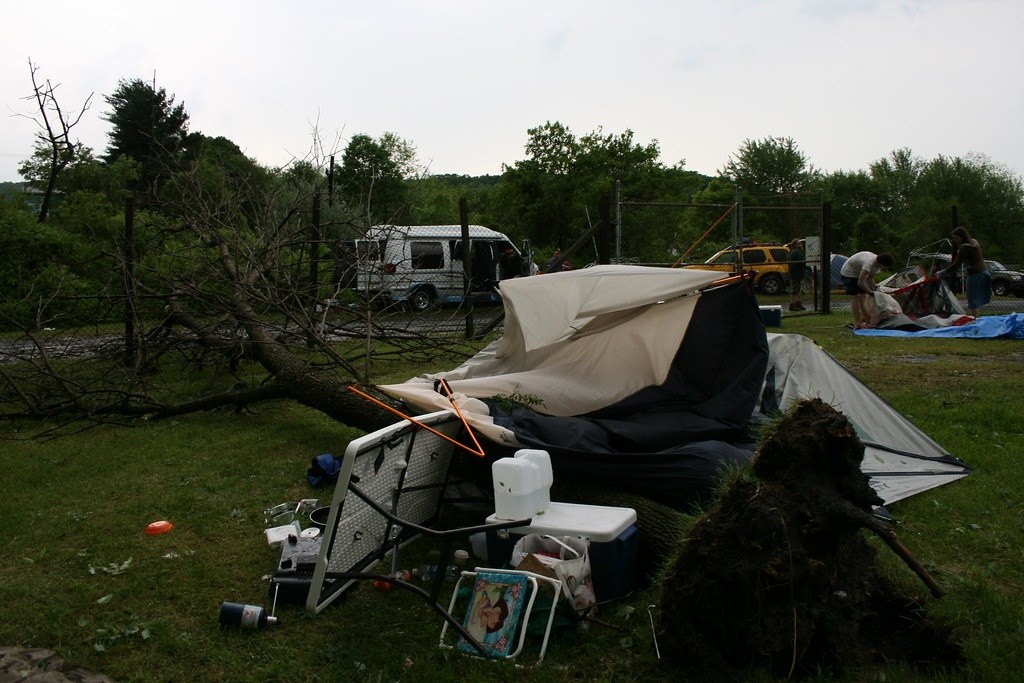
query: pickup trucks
[682,243,794,297]
[906,253,1024,296]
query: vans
[356,223,542,312]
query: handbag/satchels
[511,532,599,622]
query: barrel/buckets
[493,449,554,522]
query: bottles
[442,549,471,586]
[372,570,411,590]
[219,602,277,629]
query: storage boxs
[486,502,643,605]
[758,305,783,328]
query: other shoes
[789,303,801,311]
[796,301,806,310]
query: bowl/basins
[309,506,331,532]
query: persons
[841,251,894,329]
[788,238,822,312]
[546,250,573,273]
[498,244,520,307]
[936,227,993,318]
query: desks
[305,409,532,662]
